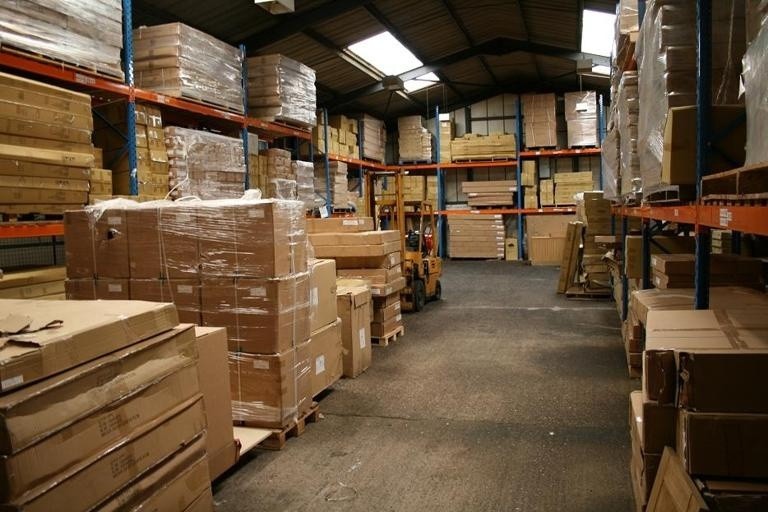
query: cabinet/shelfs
[334,114,384,189]
[138,22,248,187]
[254,105,330,190]
[1,4,136,189]
[388,136,603,216]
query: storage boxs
[582,1,767,511]
[310,218,384,259]
[442,191,612,300]
[65,270,345,429]
[1,299,215,512]
[343,288,374,378]
[63,199,309,267]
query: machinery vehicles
[363,171,442,312]
